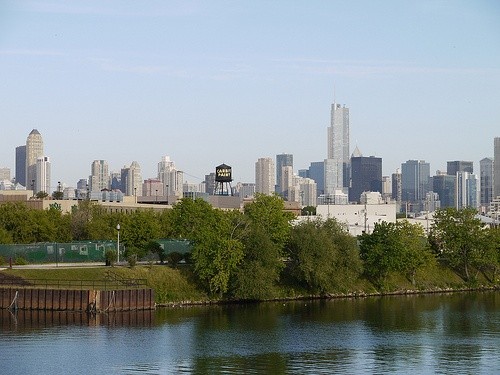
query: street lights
[58,181,60,199]
[166,184,168,203]
[31,180,35,193]
[135,187,136,204]
[156,189,158,204]
[116,224,120,263]
